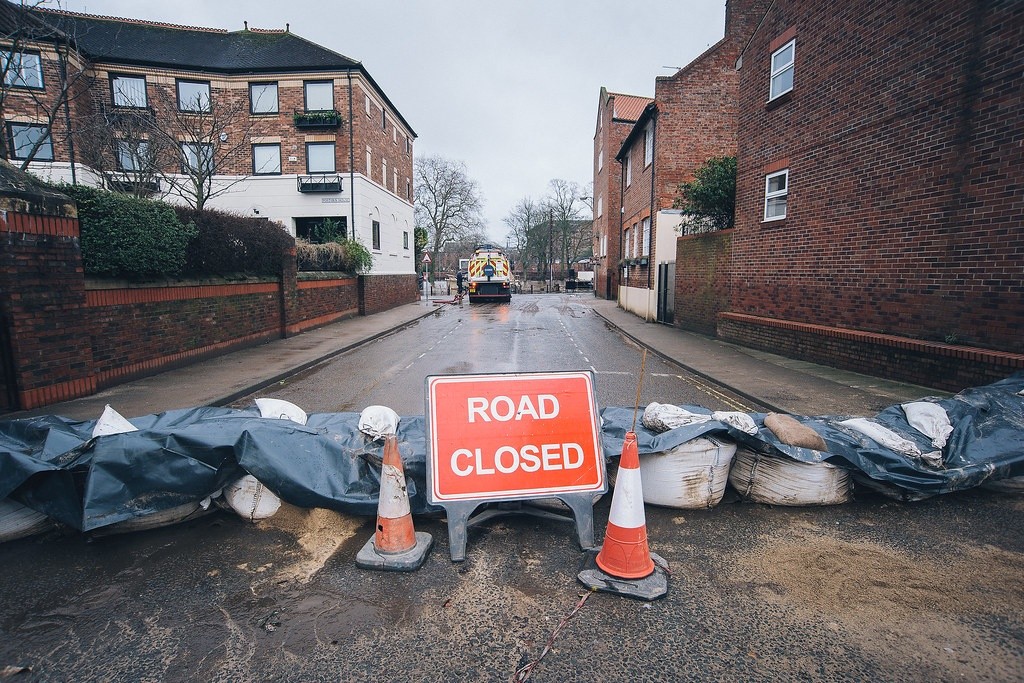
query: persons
[457,269,464,299]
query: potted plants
[292,111,341,126]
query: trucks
[466,245,514,303]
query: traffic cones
[354,434,435,571]
[576,430,673,601]
